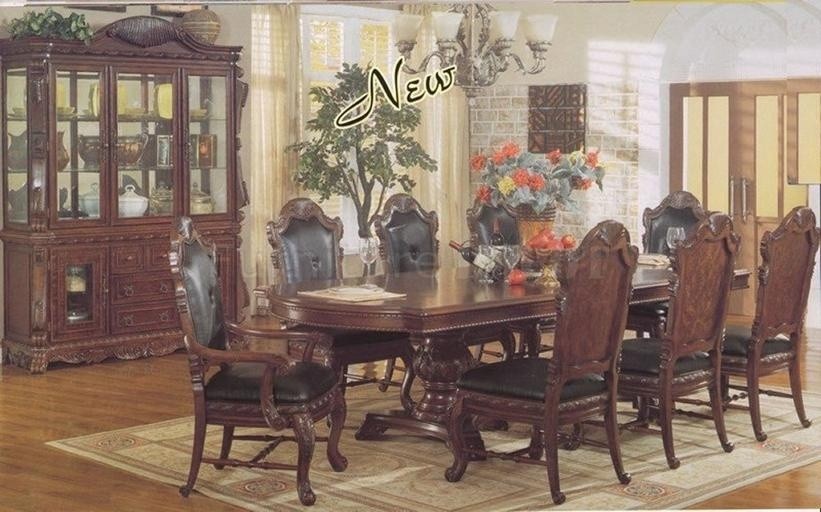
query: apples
[563,234,576,249]
[508,269,525,286]
[528,234,548,249]
[547,240,565,249]
[539,227,556,241]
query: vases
[517,202,557,273]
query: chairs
[656,206,821,441]
[466,198,555,361]
[167,216,348,505]
[445,221,631,504]
[637,192,707,338]
[375,195,517,392]
[565,212,736,470]
[266,198,418,427]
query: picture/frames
[151,4,207,18]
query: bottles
[448,239,505,280]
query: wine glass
[358,237,379,289]
[503,245,521,283]
[666,228,685,271]
[477,245,494,284]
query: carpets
[45,375,821,512]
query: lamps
[394,0,559,108]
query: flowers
[469,142,607,218]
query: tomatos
[508,267,530,289]
[529,235,552,249]
[544,229,556,236]
[549,239,561,252]
[564,236,577,249]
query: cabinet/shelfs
[0,16,251,375]
